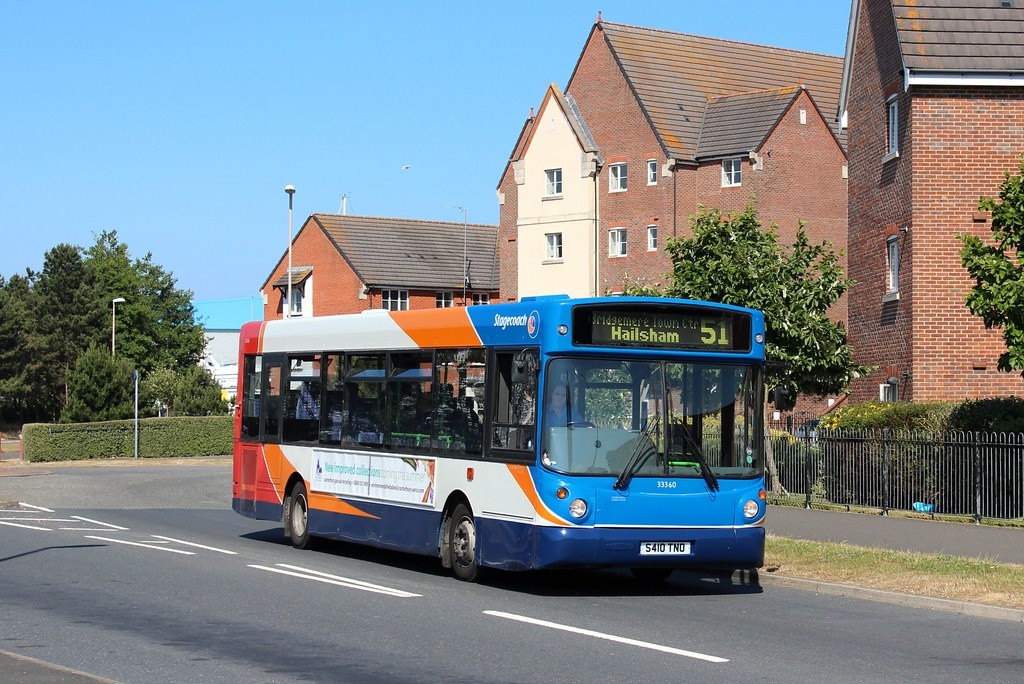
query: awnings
[344,368,433,382]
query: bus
[230,292,795,583]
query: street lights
[285,184,297,319]
[112,297,126,364]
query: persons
[543,381,588,427]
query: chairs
[252,376,471,447]
